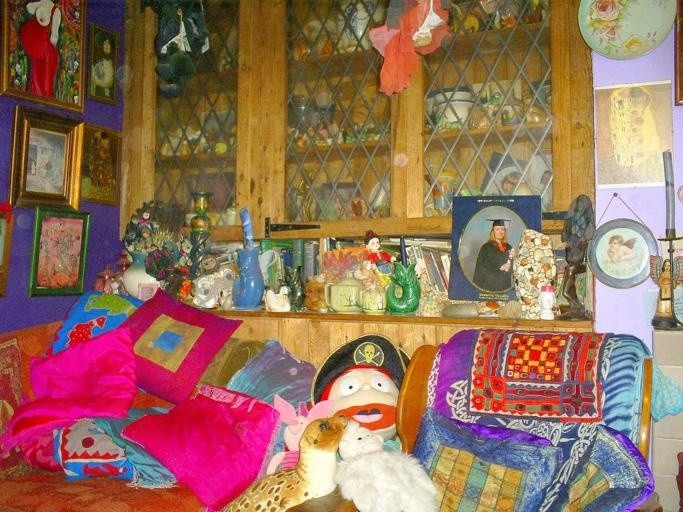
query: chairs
[386,331,654,511]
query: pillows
[1,337,29,478]
[2,325,137,453]
[228,341,318,476]
[22,403,179,489]
[117,287,244,403]
[121,383,280,509]
[50,289,144,356]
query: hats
[486,218,511,226]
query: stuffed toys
[220,333,442,512]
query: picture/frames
[3,2,123,296]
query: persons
[19,0,81,101]
[362,228,396,286]
[654,259,674,318]
[495,172,535,198]
[472,219,515,292]
[559,195,597,314]
[607,235,636,262]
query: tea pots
[324,270,388,316]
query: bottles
[538,286,556,321]
[226,199,236,225]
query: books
[205,236,452,298]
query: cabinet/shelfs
[130,0,595,251]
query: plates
[578,0,680,60]
